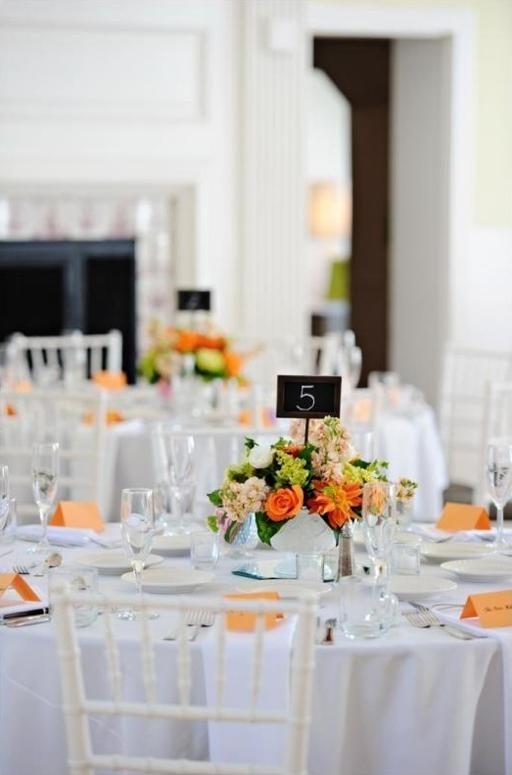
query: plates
[0,518,511,646]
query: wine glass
[119,488,156,621]
[34,442,61,566]
[158,433,195,538]
[483,446,511,550]
[362,485,398,628]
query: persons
[301,105,356,369]
